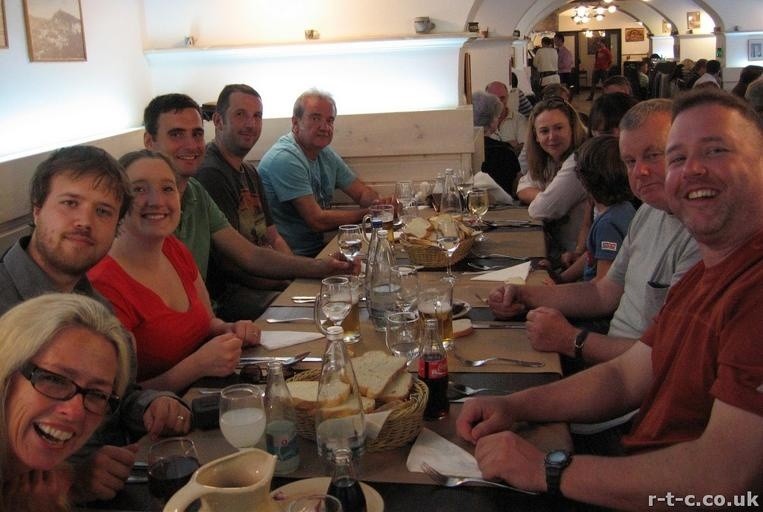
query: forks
[453,353,546,369]
[475,293,494,302]
[473,249,529,258]
[420,461,537,497]
[266,316,328,325]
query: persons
[540,134,635,284]
[728,67,761,111]
[585,39,613,102]
[541,83,588,123]
[484,81,531,181]
[257,91,402,262]
[0,295,133,510]
[590,87,636,132]
[489,96,706,366]
[510,74,533,118]
[143,94,361,319]
[691,61,721,88]
[517,98,591,236]
[534,38,560,90]
[456,88,763,511]
[602,75,630,92]
[85,150,262,392]
[195,84,298,312]
[469,92,521,201]
[684,58,707,92]
[632,62,649,94]
[2,147,133,326]
[555,33,574,101]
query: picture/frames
[0,0,10,51]
[748,38,763,61]
[23,0,88,63]
[624,27,645,42]
[687,11,700,30]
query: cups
[218,383,266,450]
[389,264,419,322]
[437,221,462,283]
[313,283,362,344]
[395,181,412,214]
[146,436,199,500]
[337,224,363,287]
[319,276,354,325]
[417,279,456,350]
[455,168,474,216]
[386,312,423,368]
[471,189,489,226]
[362,204,395,246]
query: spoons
[447,379,517,397]
[469,262,512,271]
[469,322,530,330]
[241,351,311,367]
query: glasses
[19,358,122,417]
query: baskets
[286,366,428,450]
[400,230,474,269]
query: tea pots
[161,448,279,512]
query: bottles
[365,219,396,319]
[264,362,300,479]
[316,326,367,466]
[418,319,450,422]
[370,230,404,332]
[326,448,368,512]
[440,168,462,215]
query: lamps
[570,4,592,26]
[592,0,617,26]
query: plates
[416,294,472,320]
[268,475,385,512]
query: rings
[252,331,257,335]
[177,415,184,419]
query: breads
[457,223,471,237]
[280,381,350,407]
[378,372,412,402]
[320,396,375,419]
[403,217,433,241]
[452,319,474,338]
[340,350,407,399]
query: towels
[471,261,533,285]
[259,330,325,351]
[406,426,483,478]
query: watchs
[574,329,590,361]
[544,446,572,496]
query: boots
[586,91,596,101]
